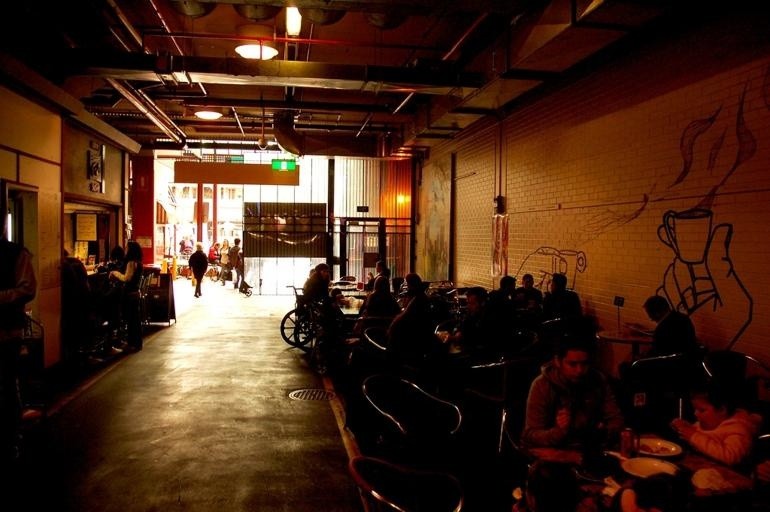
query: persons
[178,234,255,299]
[0,210,38,512]
[58,239,145,360]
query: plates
[639,437,682,456]
[576,451,627,481]
[621,457,678,478]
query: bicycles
[204,256,222,282]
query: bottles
[620,424,639,458]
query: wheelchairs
[281,285,333,353]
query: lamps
[194,107,224,121]
[235,25,277,61]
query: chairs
[304,273,764,511]
